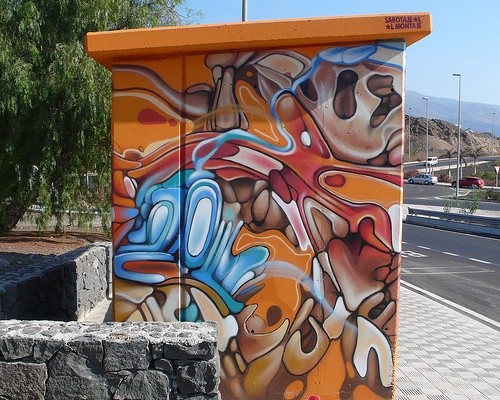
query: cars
[408,174,438,185]
[450,176,485,189]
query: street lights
[492,113,496,157]
[453,73,462,200]
[408,107,413,162]
[422,97,428,174]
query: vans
[424,157,438,166]
